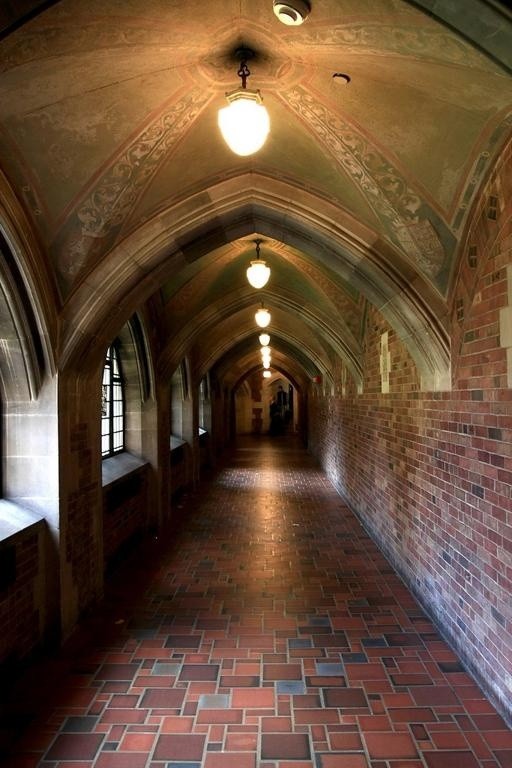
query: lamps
[246,237,273,331]
[216,45,269,160]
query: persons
[277,386,286,416]
